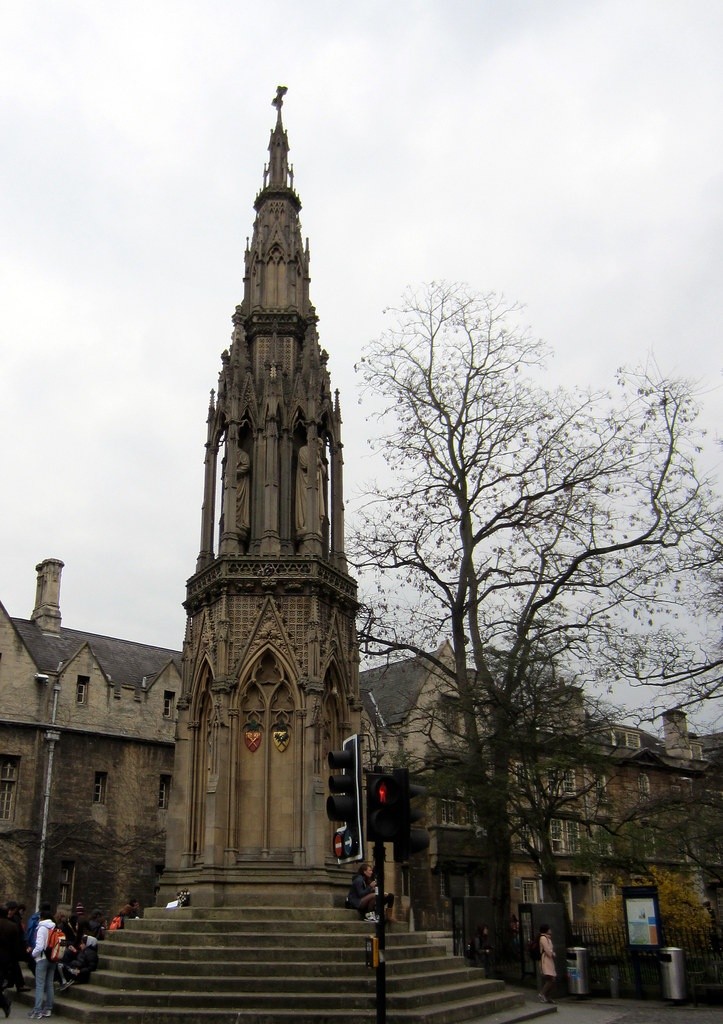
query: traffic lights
[393,768,429,863]
[326,733,365,865]
[365,772,401,842]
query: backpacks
[43,927,67,963]
[109,916,122,929]
[530,935,548,961]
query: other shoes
[364,913,379,923]
[3,999,12,1018]
[60,978,75,990]
[42,1009,52,1017]
[27,1011,42,1019]
[370,911,379,919]
[536,993,547,1003]
[548,999,556,1003]
[16,986,31,992]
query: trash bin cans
[566,946,591,1000]
[659,947,687,1007]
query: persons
[536,923,557,1004]
[25,901,59,1019]
[0,900,30,1018]
[55,904,103,991]
[102,898,143,929]
[346,864,398,923]
[218,436,250,529]
[296,437,326,533]
[474,925,496,978]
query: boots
[386,908,397,923]
